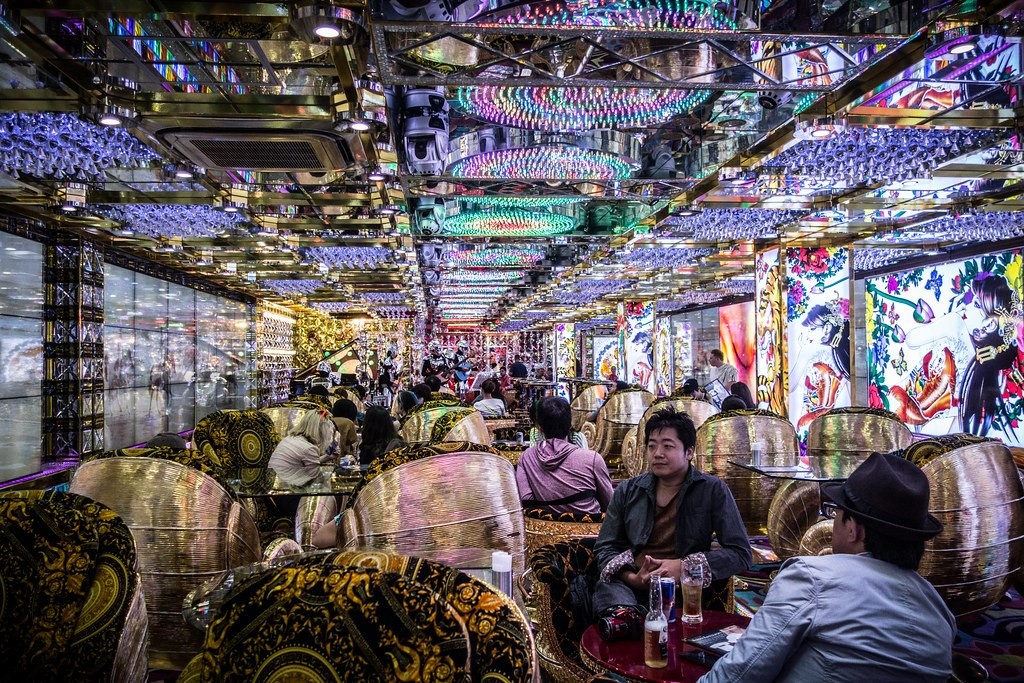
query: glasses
[822,501,843,519]
[398,390,403,403]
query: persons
[472,355,553,419]
[683,349,755,411]
[697,452,957,683]
[586,381,629,424]
[609,366,618,381]
[311,509,344,547]
[307,339,471,476]
[592,404,753,623]
[267,410,341,489]
[516,396,614,514]
[572,354,582,377]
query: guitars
[435,354,473,384]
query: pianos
[289,340,365,397]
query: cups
[681,621,705,679]
[680,558,704,624]
[752,450,761,466]
[491,569,512,600]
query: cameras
[597,605,644,642]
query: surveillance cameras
[415,196,444,296]
[401,89,450,173]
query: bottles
[644,573,668,669]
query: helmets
[428,340,442,356]
[387,348,398,358]
[457,340,469,353]
[316,361,331,374]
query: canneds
[516,432,523,445]
[656,577,676,623]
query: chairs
[0,385,1024,683]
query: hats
[820,452,944,539]
[684,379,699,395]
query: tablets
[679,624,747,658]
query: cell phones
[325,441,338,455]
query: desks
[578,606,752,683]
[572,405,597,413]
[222,466,357,498]
[726,456,868,519]
[603,415,642,425]
